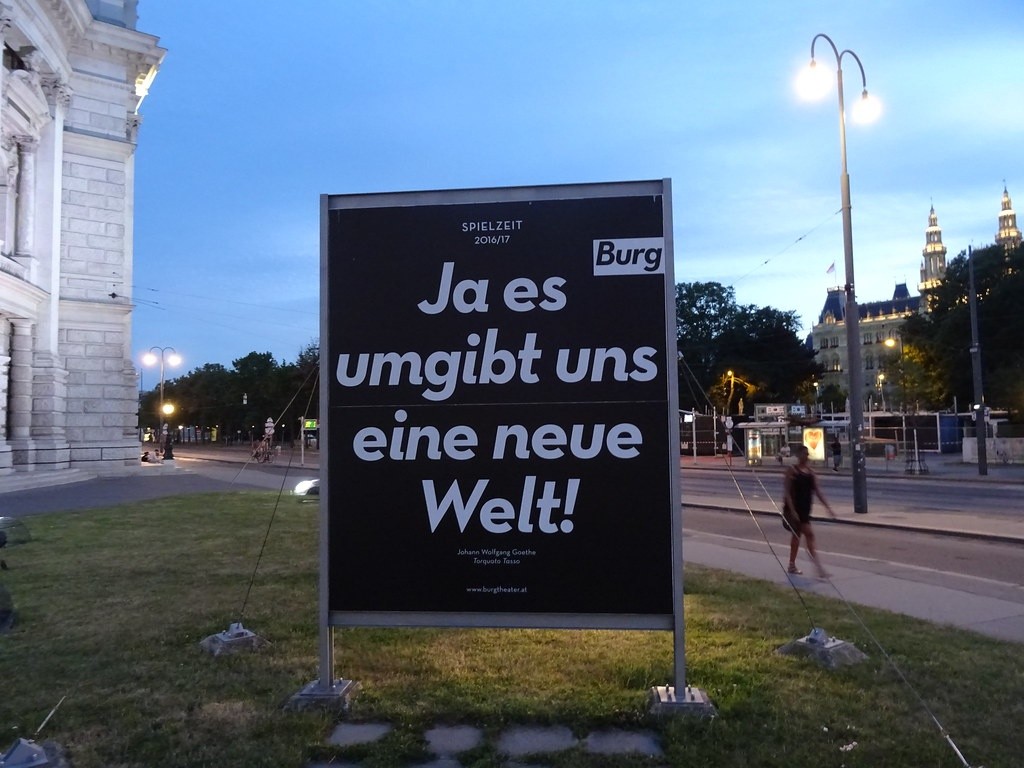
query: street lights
[886,327,909,463]
[799,33,879,515]
[145,347,180,453]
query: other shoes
[788,566,802,575]
[820,571,833,578]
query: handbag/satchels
[783,504,799,532]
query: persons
[782,445,837,579]
[141,449,165,464]
[829,436,841,472]
[252,438,262,459]
